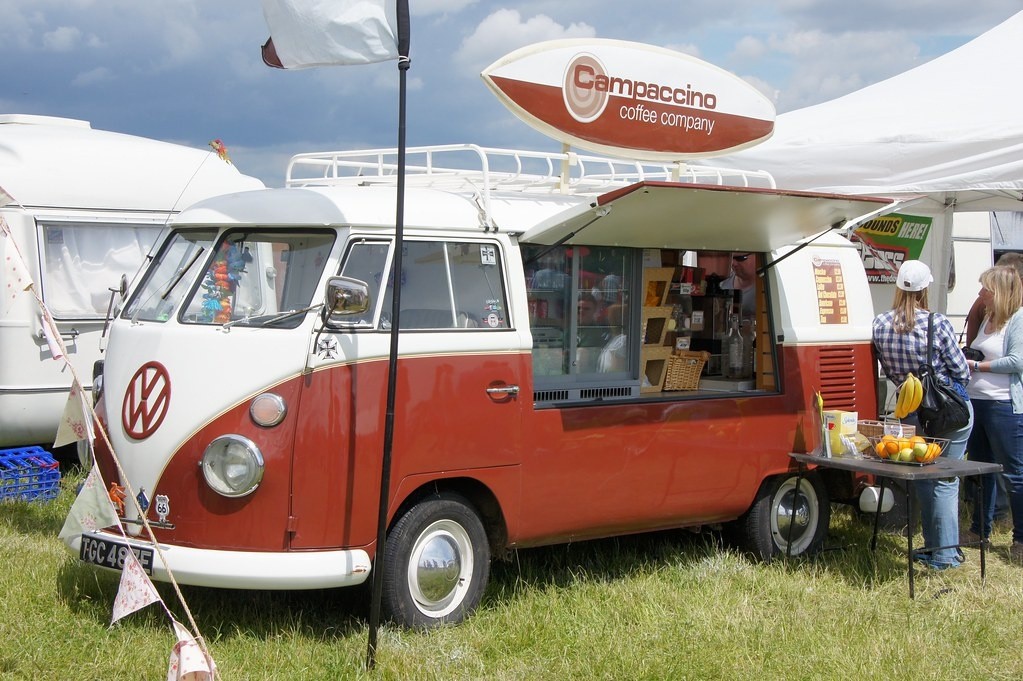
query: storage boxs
[642,267,677,393]
[0,447,61,502]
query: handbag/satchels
[917,312,970,440]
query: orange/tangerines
[876,435,942,463]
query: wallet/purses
[962,346,985,361]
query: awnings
[518,181,895,273]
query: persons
[872,253,1023,571]
[525,255,628,372]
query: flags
[261,0,400,70]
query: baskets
[662,349,711,391]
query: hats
[896,260,933,291]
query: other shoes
[959,530,990,549]
[912,547,960,570]
[1009,540,1023,561]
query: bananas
[894,372,923,419]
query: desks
[788,457,1003,603]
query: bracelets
[974,361,980,372]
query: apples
[901,448,916,462]
[889,451,901,461]
[912,442,927,456]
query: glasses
[733,253,752,261]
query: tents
[685,10,1023,214]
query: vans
[63,145,908,636]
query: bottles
[728,314,744,379]
[741,320,753,378]
[752,337,757,379]
